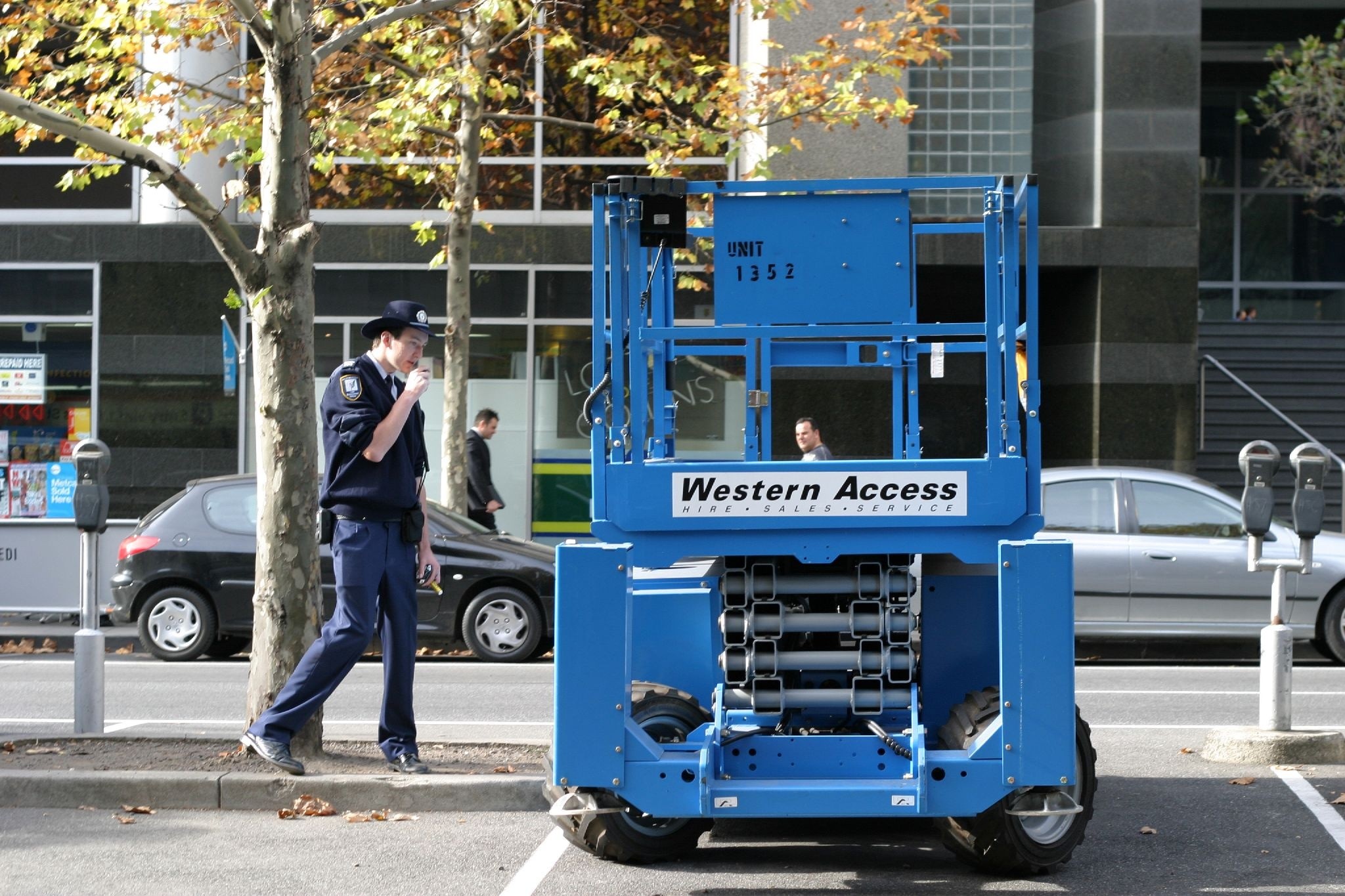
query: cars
[112,475,557,664]
[1032,466,1345,665]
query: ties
[385,375,392,392]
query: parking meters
[70,436,113,734]
[1237,439,1332,734]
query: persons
[238,301,441,776]
[794,417,836,463]
[465,409,507,534]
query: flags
[223,317,236,397]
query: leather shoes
[240,732,305,775]
[388,753,429,774]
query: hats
[362,300,437,339]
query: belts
[334,514,401,522]
